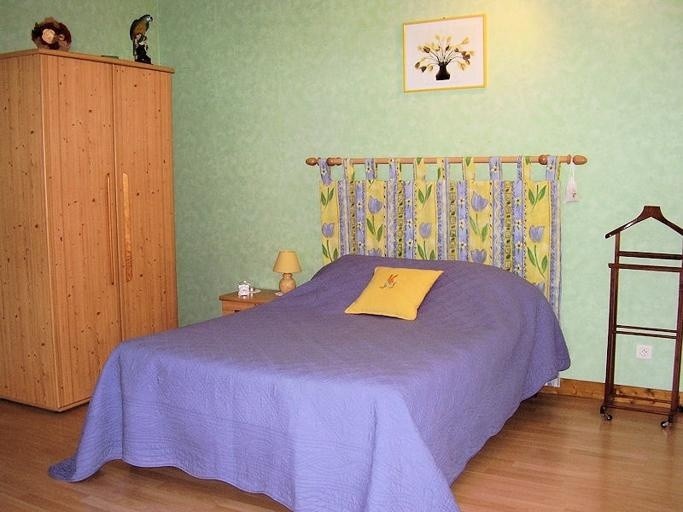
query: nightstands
[218,289,280,315]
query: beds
[47,153,588,512]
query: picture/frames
[401,13,488,93]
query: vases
[435,64,450,80]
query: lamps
[272,250,302,294]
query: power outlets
[635,344,651,359]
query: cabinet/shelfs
[0,47,179,414]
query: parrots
[129,14,154,56]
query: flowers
[413,33,476,73]
